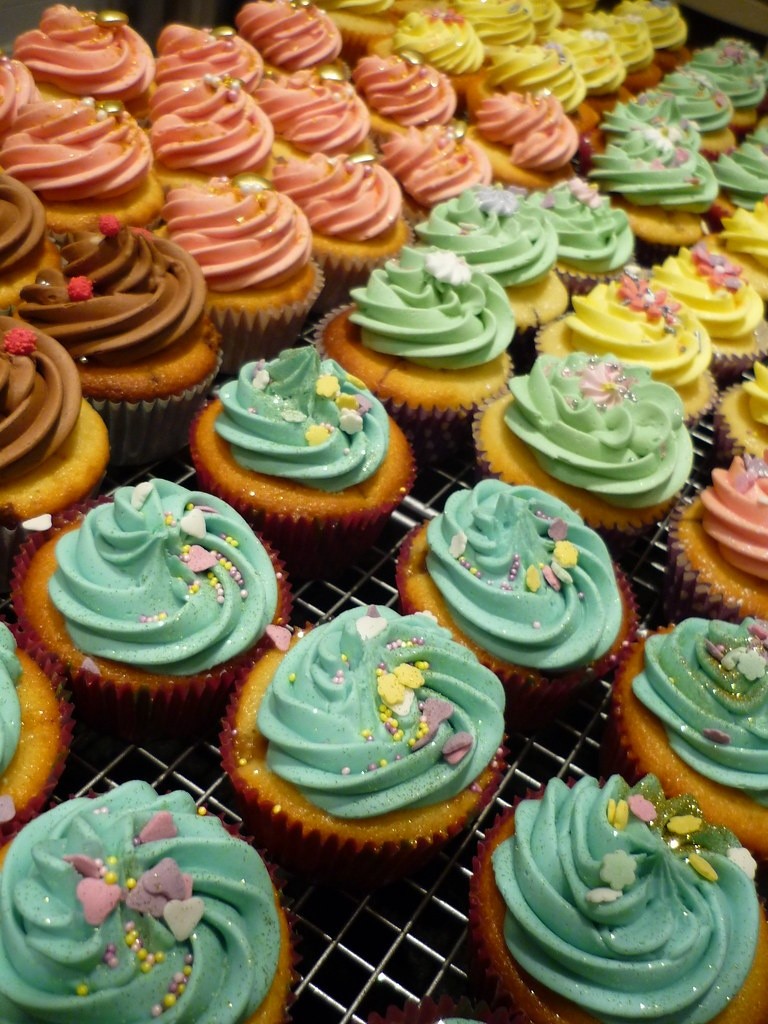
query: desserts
[0,0,768,1024]
